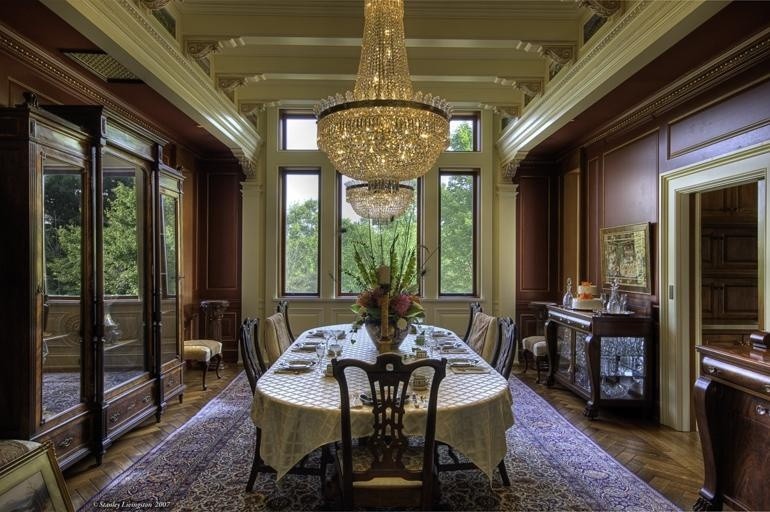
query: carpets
[75,368,684,512]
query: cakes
[571,280,605,310]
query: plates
[428,329,492,376]
[359,388,409,403]
[272,328,323,377]
[408,382,430,390]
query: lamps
[345,183,415,226]
[312,0,454,194]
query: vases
[364,317,411,350]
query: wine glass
[315,328,346,379]
[599,293,607,313]
[620,293,627,313]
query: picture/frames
[599,222,652,295]
[0,440,75,512]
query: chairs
[240,300,334,494]
[521,326,564,384]
[434,302,516,486]
[331,352,447,512]
[183,300,229,390]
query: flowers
[340,206,441,344]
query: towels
[339,392,363,409]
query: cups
[413,375,430,387]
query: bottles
[563,277,573,309]
[401,334,427,364]
[607,278,621,314]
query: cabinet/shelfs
[545,305,659,426]
